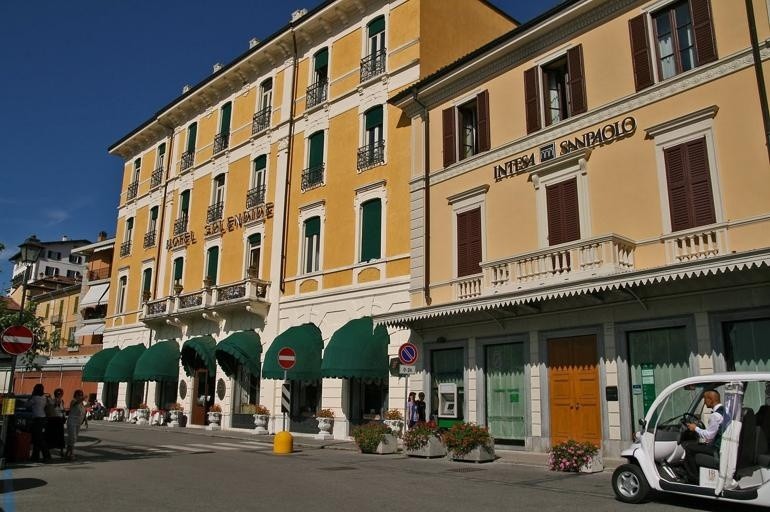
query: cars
[0,392,67,432]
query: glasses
[58,393,61,396]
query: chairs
[696,405,770,467]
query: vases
[577,450,603,473]
[136,409,148,425]
[207,412,221,430]
[449,437,495,463]
[254,414,269,434]
[167,411,183,427]
[316,418,333,440]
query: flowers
[139,403,148,409]
[209,405,221,412]
[254,407,270,415]
[316,409,335,418]
[546,438,599,473]
[351,421,496,456]
[169,403,184,411]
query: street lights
[7,234,46,393]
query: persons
[47,389,66,461]
[65,390,88,461]
[685,389,730,485]
[407,392,418,431]
[91,400,102,413]
[416,392,426,423]
[25,384,48,463]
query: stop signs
[1,325,33,356]
[278,347,296,370]
